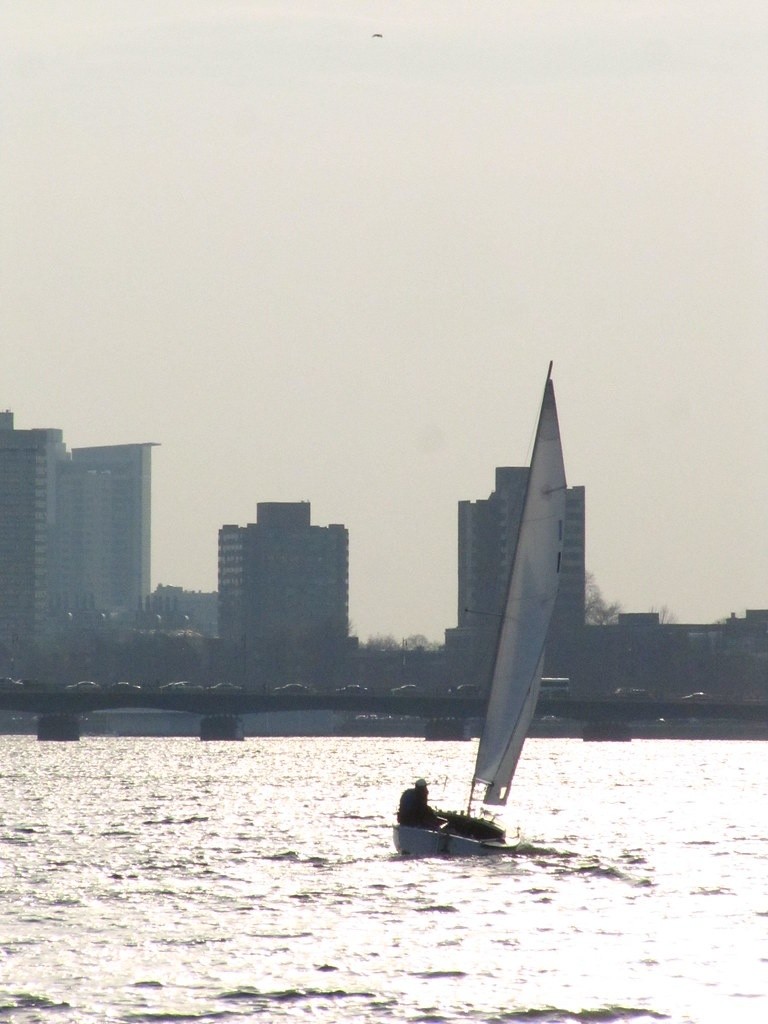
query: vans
[0,678,24,690]
[16,679,39,685]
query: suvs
[447,684,481,698]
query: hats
[415,779,430,787]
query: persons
[400,779,448,831]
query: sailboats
[393,362,569,855]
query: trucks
[614,688,651,700]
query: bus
[539,677,571,700]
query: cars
[159,681,204,692]
[107,681,142,690]
[683,691,715,704]
[336,685,368,697]
[65,682,103,693]
[392,685,418,695]
[272,682,313,694]
[205,682,246,693]
